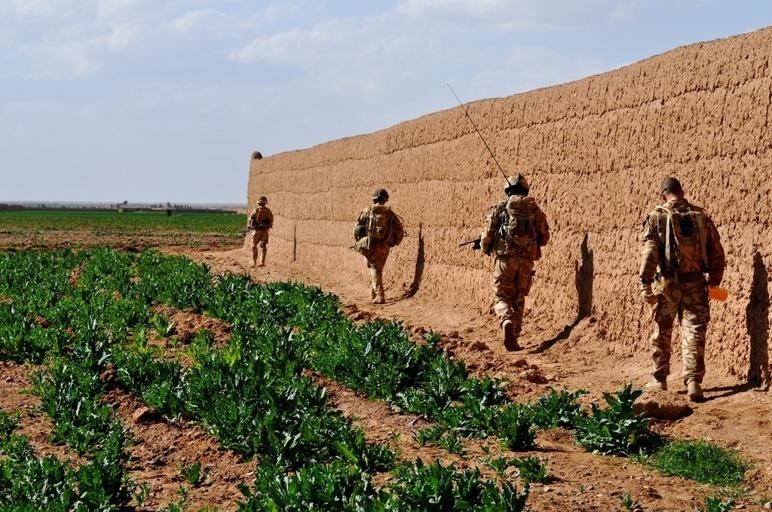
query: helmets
[504,176,529,196]
[372,187,389,200]
[257,196,268,204]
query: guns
[459,239,481,249]
[241,224,268,235]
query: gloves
[641,283,658,304]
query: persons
[639,178,728,403]
[352,188,404,304]
[479,173,550,352]
[247,196,273,267]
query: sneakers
[249,263,265,267]
[501,320,516,351]
[370,294,385,303]
[687,381,705,402]
[646,379,667,390]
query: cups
[708,286,728,301]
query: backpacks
[505,196,538,247]
[367,205,389,240]
[256,207,270,225]
[657,204,709,272]
